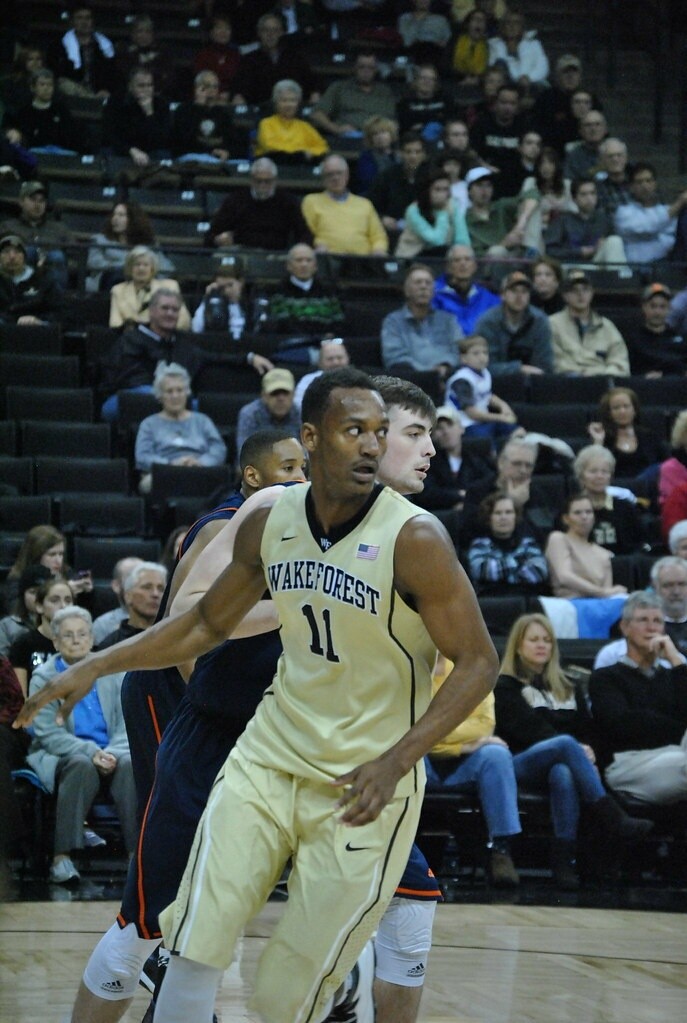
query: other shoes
[2,841,39,882]
[82,828,107,847]
[492,853,519,885]
[48,860,81,883]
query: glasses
[252,175,274,185]
[217,281,233,294]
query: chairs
[0,2,686,911]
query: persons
[0,1,687,1023]
[13,364,500,1022]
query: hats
[464,166,496,191]
[435,407,461,426]
[502,270,534,294]
[18,181,45,201]
[262,368,295,394]
[560,267,595,286]
[556,54,584,75]
[641,282,672,302]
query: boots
[585,795,655,852]
[545,845,591,892]
